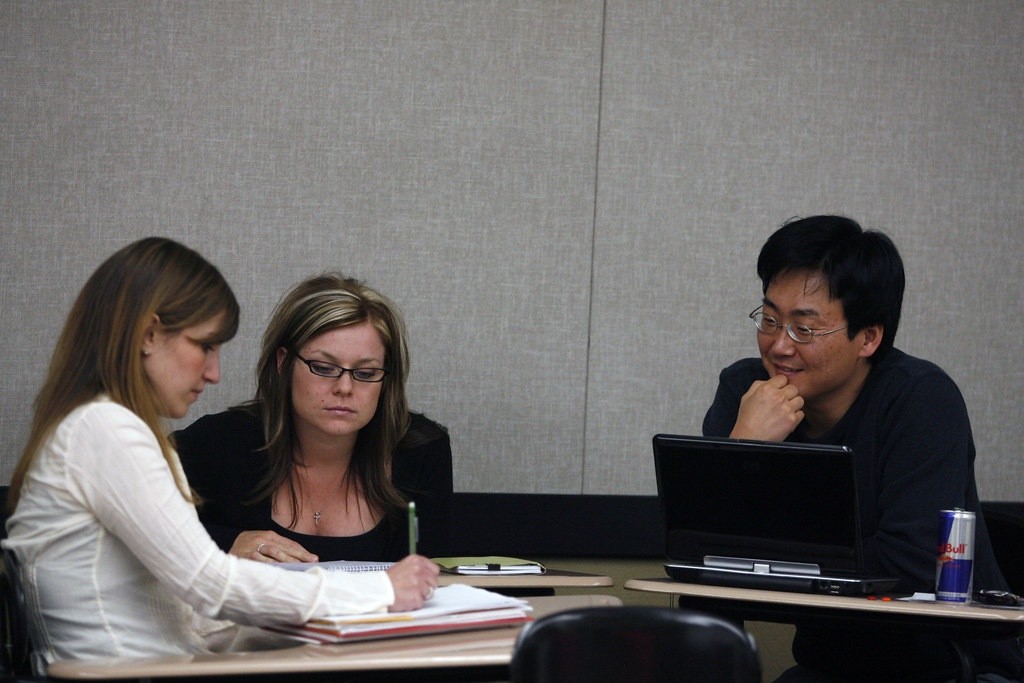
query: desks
[436,566,616,597]
[623,576,1024,621]
[46,595,624,683]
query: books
[432,556,546,576]
[256,560,538,645]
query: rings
[425,586,433,600]
[253,541,269,553]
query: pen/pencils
[409,501,416,555]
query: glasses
[749,304,847,343]
[296,353,387,382]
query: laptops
[650,432,918,598]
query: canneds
[935,507,976,605]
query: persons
[2,236,440,678]
[164,275,455,561]
[702,212,1002,591]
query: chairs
[0,479,1024,683]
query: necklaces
[291,450,353,528]
